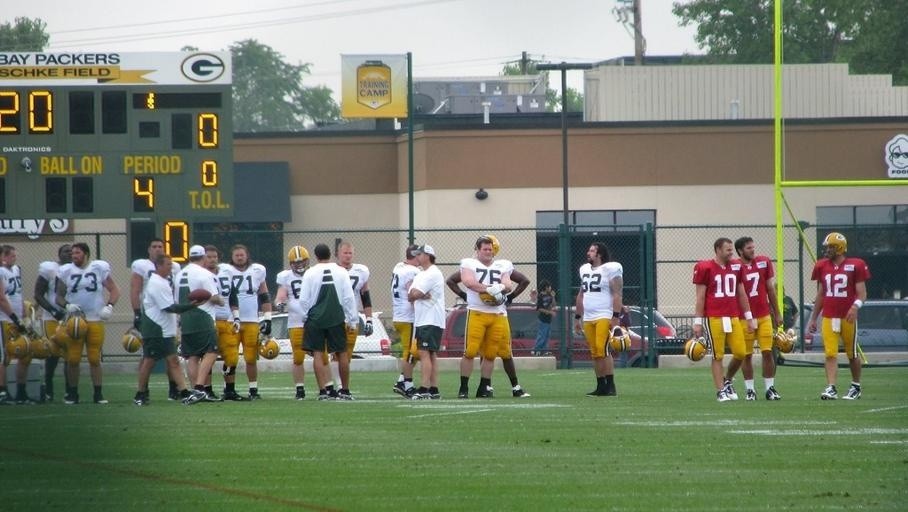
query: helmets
[822,232,848,258]
[684,337,708,362]
[121,330,144,353]
[257,333,280,360]
[481,287,506,306]
[609,325,632,354]
[289,245,310,262]
[485,236,500,257]
[773,330,796,353]
[3,314,88,360]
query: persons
[734,238,783,402]
[129,239,374,402]
[447,235,532,397]
[0,241,120,403]
[408,245,447,397]
[811,231,870,398]
[692,237,755,402]
[459,235,514,399]
[573,243,623,396]
[391,246,425,397]
[530,281,556,357]
[774,284,799,331]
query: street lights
[535,61,595,225]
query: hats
[411,244,436,257]
[189,245,206,257]
[406,245,424,259]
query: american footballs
[188,289,211,302]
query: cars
[554,304,676,341]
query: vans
[791,300,907,353]
[436,302,656,368]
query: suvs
[178,310,393,358]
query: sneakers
[512,389,531,398]
[717,377,862,404]
[585,385,604,397]
[595,388,616,398]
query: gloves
[232,318,242,333]
[101,306,113,320]
[52,309,64,321]
[65,302,81,313]
[364,321,374,337]
[133,316,141,330]
[260,320,272,336]
[277,303,287,314]
[16,322,26,332]
